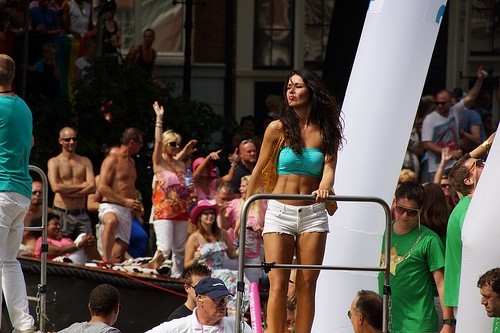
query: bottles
[133,192,142,211]
[185,167,192,189]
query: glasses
[133,139,144,147]
[200,211,216,215]
[62,136,78,142]
[434,100,449,106]
[347,310,360,319]
[32,189,43,195]
[242,138,253,144]
[468,159,485,173]
[395,197,422,218]
[119,304,122,310]
[167,141,183,147]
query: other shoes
[12,328,35,333]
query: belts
[52,206,86,216]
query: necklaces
[0,90,13,94]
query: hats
[192,157,217,178]
[189,200,219,225]
[195,277,235,299]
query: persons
[348,65,500,333]
[125,30,166,88]
[0,54,33,333]
[0,0,122,98]
[246,70,347,333]
[27,101,304,333]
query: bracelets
[155,124,163,127]
[154,121,163,123]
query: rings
[313,192,317,196]
